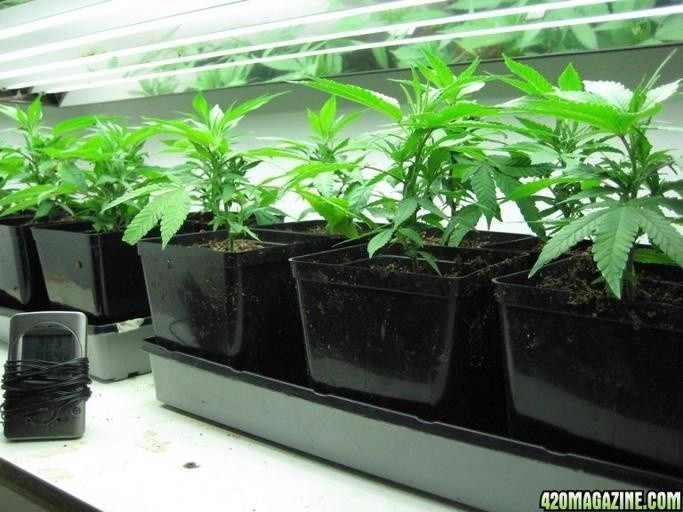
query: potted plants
[0,43,682,479]
